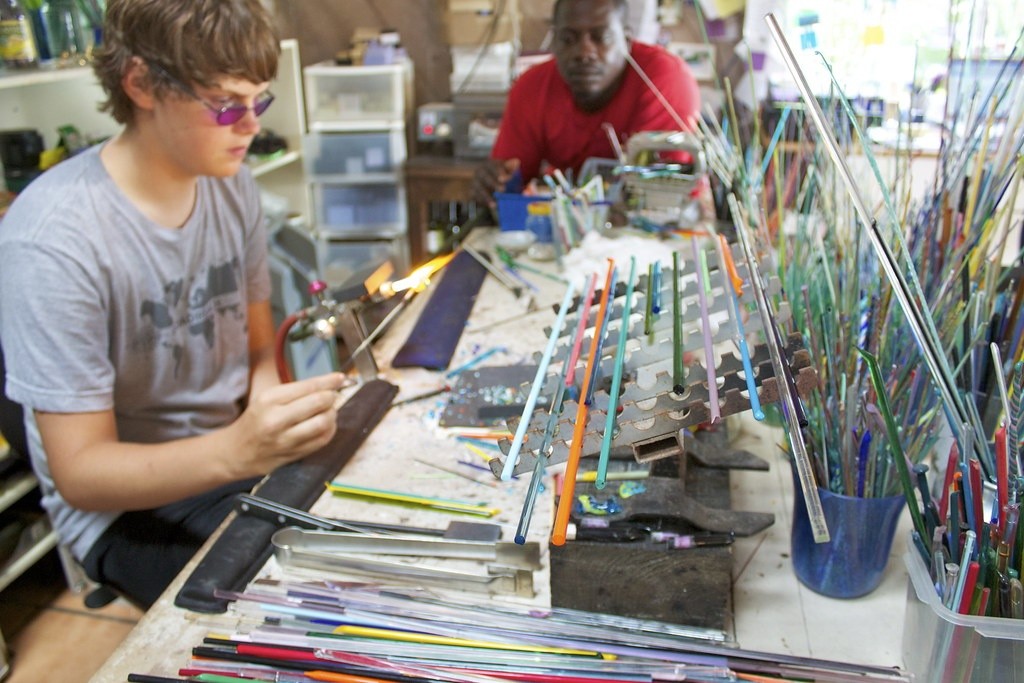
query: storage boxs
[902,530,1024,683]
[445,0,521,46]
[493,173,553,231]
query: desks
[759,135,938,209]
[400,154,485,267]
[88,227,934,683]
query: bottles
[0,0,41,71]
[39,0,79,70]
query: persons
[471,0,701,210]
[0,0,346,613]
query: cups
[790,474,908,598]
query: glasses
[144,58,275,126]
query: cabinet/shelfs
[0,38,319,593]
[299,57,416,286]
